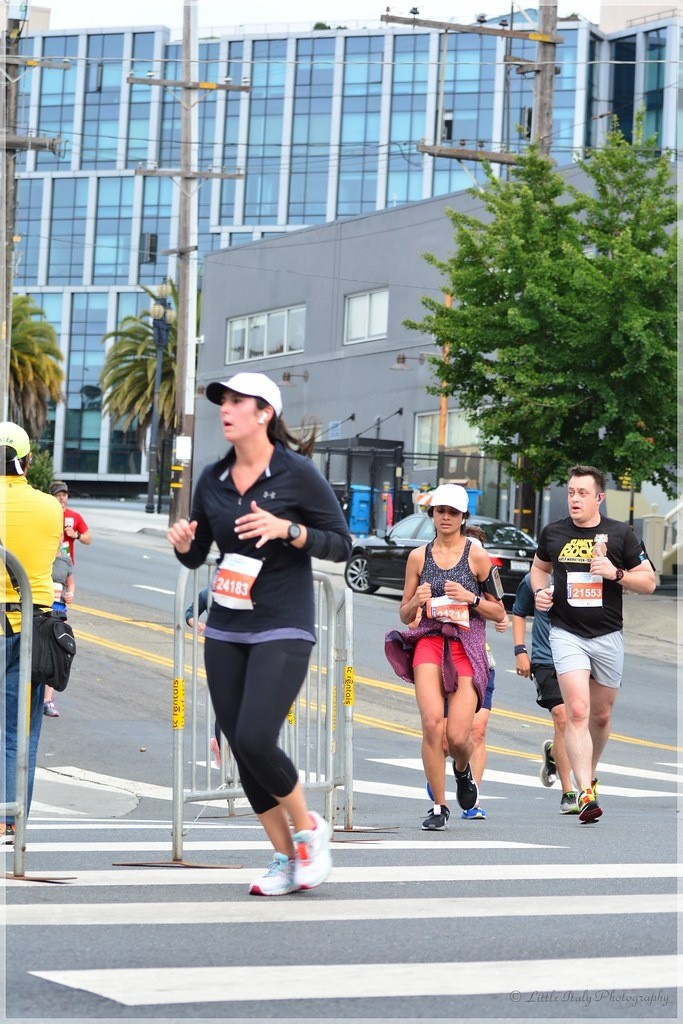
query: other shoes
[211,739,221,767]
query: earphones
[258,412,268,424]
[432,517,434,522]
[598,495,601,501]
[461,519,465,524]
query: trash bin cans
[465,487,483,516]
[348,484,379,538]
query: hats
[205,372,282,420]
[0,421,31,475]
[50,481,69,495]
[430,483,469,512]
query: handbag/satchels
[30,610,76,692]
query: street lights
[145,275,178,514]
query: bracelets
[69,591,74,597]
[514,645,528,656]
[77,533,81,539]
[534,588,543,601]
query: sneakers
[452,761,478,811]
[560,790,580,814]
[577,788,602,822]
[4,823,16,844]
[291,810,334,889]
[426,780,436,801]
[44,701,60,717]
[541,739,558,787]
[462,807,485,819]
[591,779,601,803]
[249,851,297,896]
[421,804,451,831]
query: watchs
[468,594,480,607]
[281,520,301,547]
[613,568,623,583]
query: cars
[344,510,538,612]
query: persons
[166,372,352,896]
[532,464,656,823]
[0,421,64,848]
[49,480,91,566]
[399,483,510,831]
[187,558,235,769]
[42,530,75,718]
[511,569,580,815]
